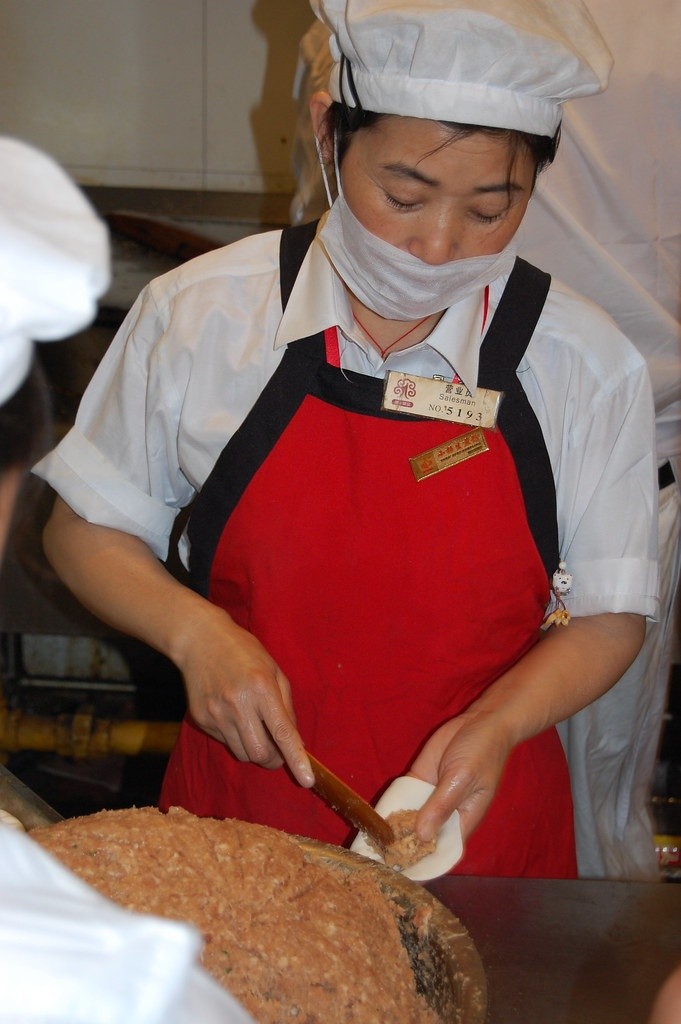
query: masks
[314,128,516,321]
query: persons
[0,134,681,1024]
[28,0,658,880]
[300,1,681,879]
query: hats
[310,0,616,140]
[0,136,112,405]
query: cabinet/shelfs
[0,0,316,192]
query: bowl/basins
[288,834,489,1024]
